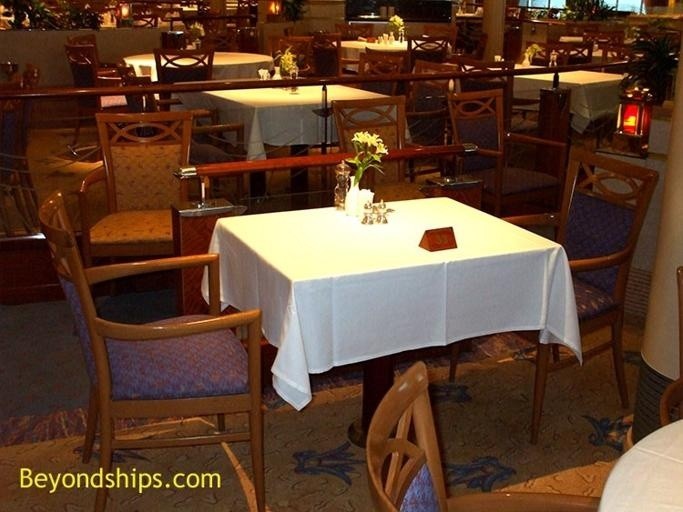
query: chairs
[492,142,659,441]
[365,360,602,512]
[36,188,269,512]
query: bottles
[334,162,350,212]
[289,68,297,92]
[377,32,394,45]
[360,200,386,226]
[548,51,557,73]
